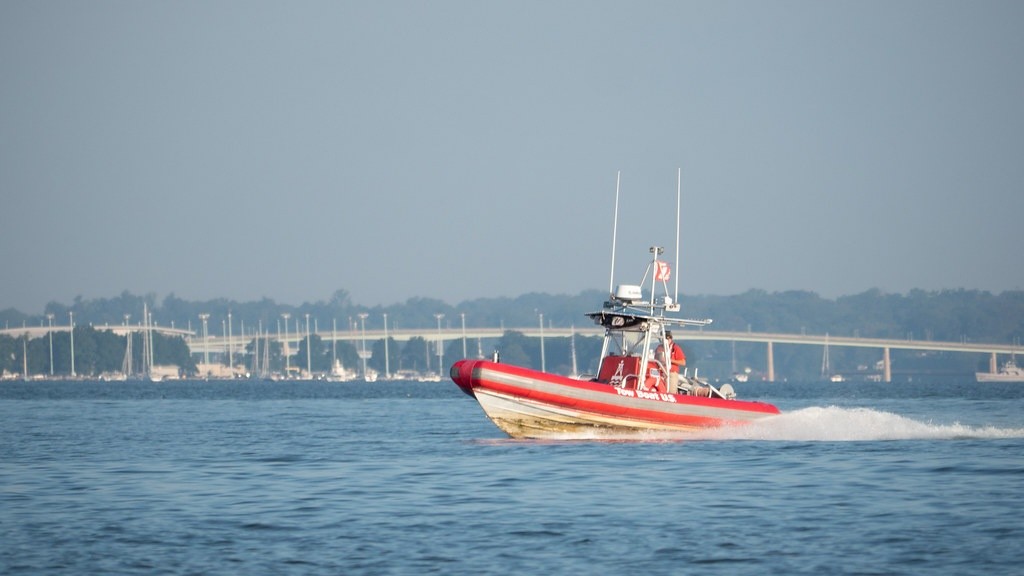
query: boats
[447,169,783,442]
[975,360,1024,383]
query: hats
[660,331,672,339]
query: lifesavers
[642,375,668,394]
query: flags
[655,260,671,282]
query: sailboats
[0,302,592,381]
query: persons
[654,330,686,394]
